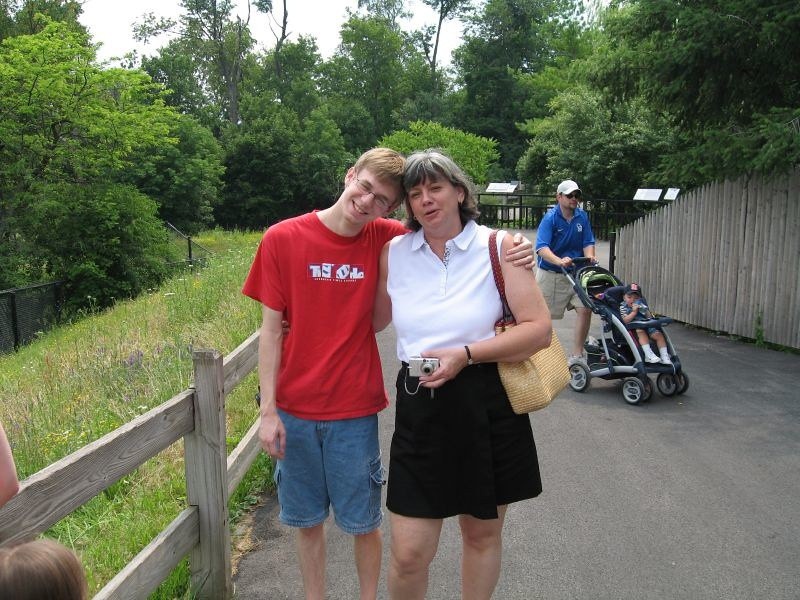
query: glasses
[562,192,580,199]
[352,175,391,210]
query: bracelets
[464,346,473,367]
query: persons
[0,540,88,600]
[241,147,536,600]
[281,151,553,600]
[534,180,599,369]
[619,282,672,365]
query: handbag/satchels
[489,228,572,415]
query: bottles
[633,299,648,315]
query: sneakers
[662,353,672,365]
[645,353,661,363]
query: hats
[556,180,583,195]
[624,283,642,298]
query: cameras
[408,357,439,377]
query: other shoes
[568,355,591,372]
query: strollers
[563,255,691,408]
